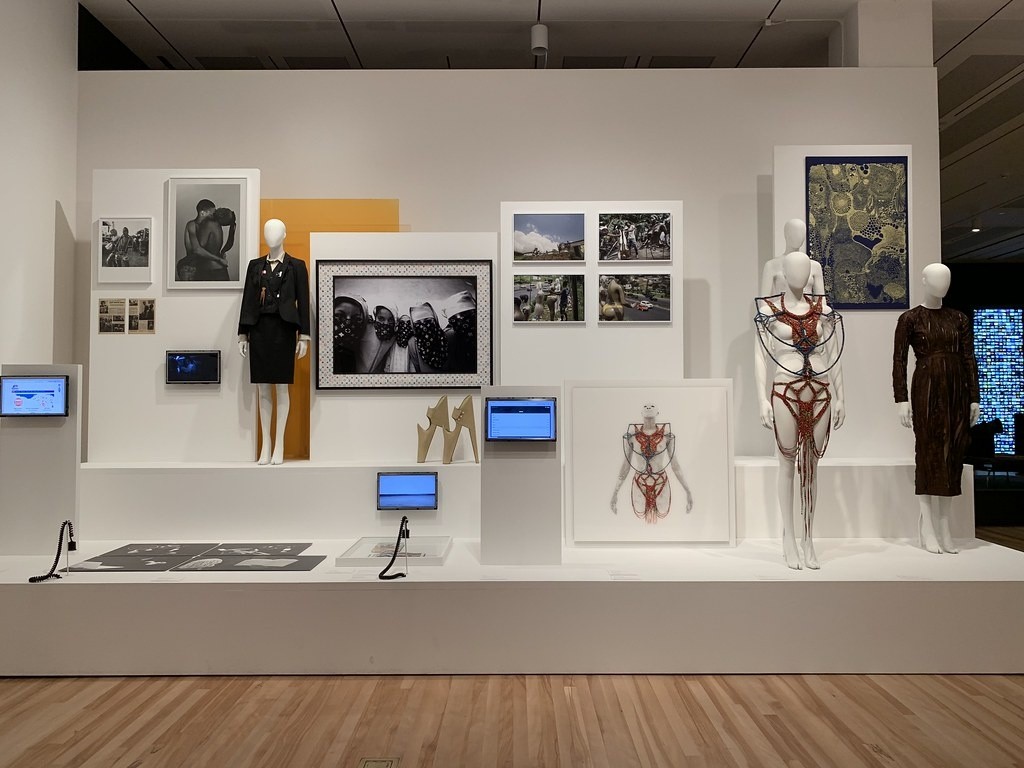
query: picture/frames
[315,259,493,389]
[804,156,910,309]
[563,378,736,547]
[98,217,153,284]
[166,349,222,384]
[514,212,673,324]
[167,177,247,289]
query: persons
[600,275,641,321]
[514,281,569,321]
[334,290,478,375]
[177,199,237,282]
[755,251,846,573]
[599,214,670,260]
[238,218,311,465]
[761,218,826,307]
[892,262,981,555]
[106,227,134,267]
[100,299,155,332]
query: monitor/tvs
[0,375,69,417]
[166,350,221,384]
[485,397,557,442]
[377,472,438,511]
